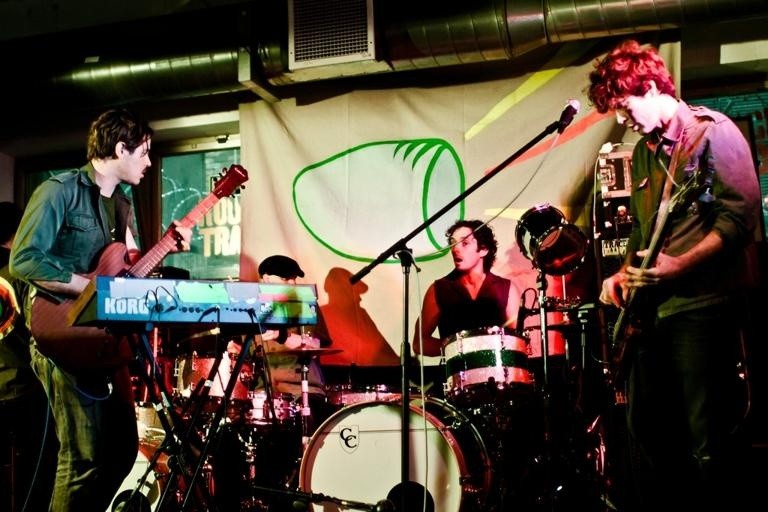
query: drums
[440,327,536,402]
[104,438,214,511]
[136,401,172,437]
[299,395,492,512]
[516,204,590,276]
[174,351,253,402]
[228,389,290,429]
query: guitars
[602,138,716,388]
[31,164,248,377]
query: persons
[8,105,194,511]
[580,41,762,512]
[220,255,332,403]
[0,200,34,402]
[412,218,535,358]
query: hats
[258,254,304,276]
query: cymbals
[263,343,341,355]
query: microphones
[339,499,395,512]
[515,293,527,337]
[558,99,580,134]
